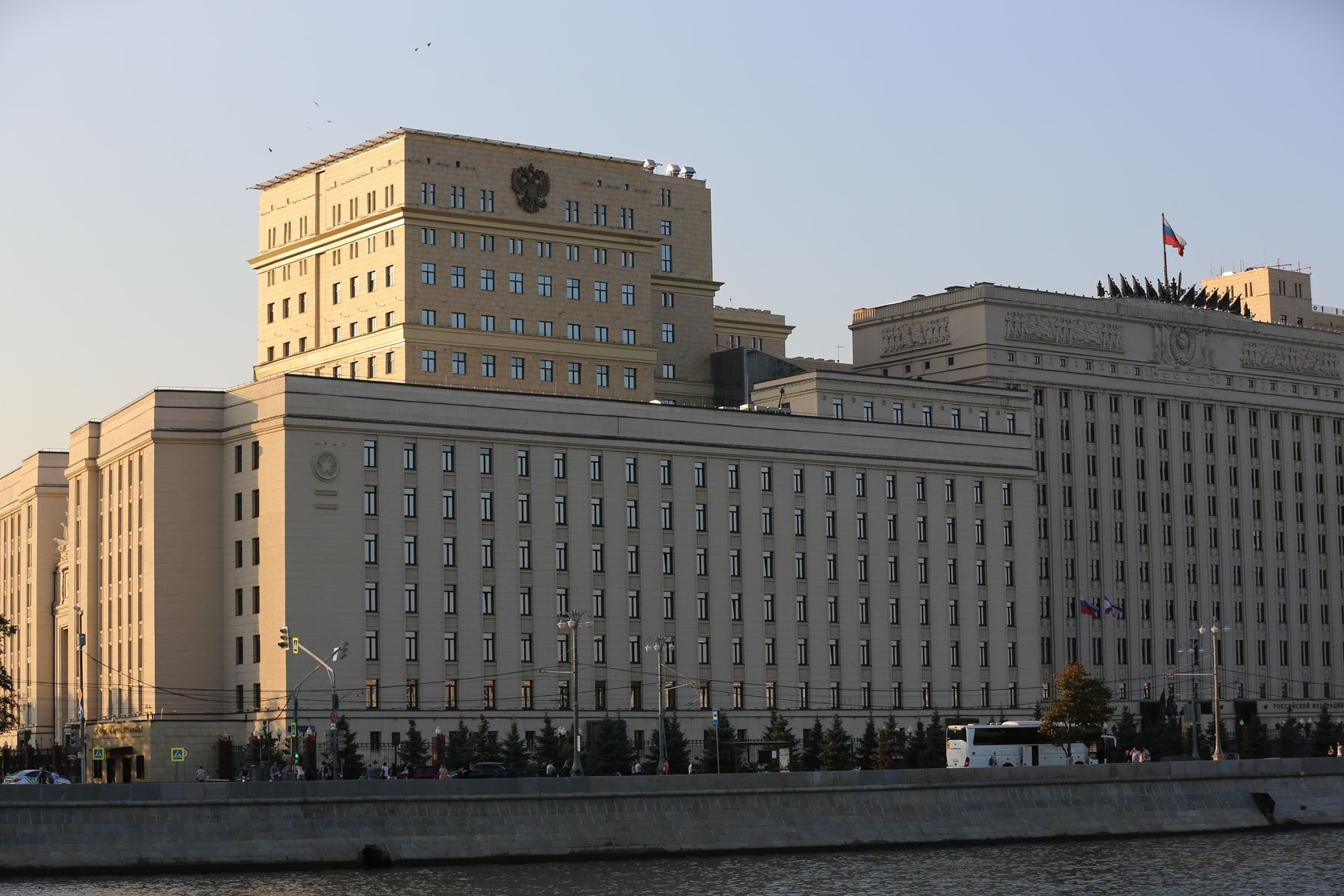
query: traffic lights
[292,752,302,766]
[282,734,292,757]
[277,626,289,651]
[338,639,349,659]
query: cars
[2,769,72,785]
[448,762,517,780]
[396,764,441,779]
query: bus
[945,721,1117,769]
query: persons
[1123,745,1151,764]
[688,759,694,774]
[662,757,669,775]
[1328,745,1335,757]
[756,761,765,773]
[632,760,643,776]
[1337,741,1344,757]
[293,761,334,782]
[545,759,571,778]
[990,751,999,767]
[439,763,448,780]
[270,762,281,781]
[240,764,251,782]
[358,761,416,782]
[196,766,207,783]
[37,767,60,784]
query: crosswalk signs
[171,747,184,762]
[92,747,104,760]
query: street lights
[556,608,595,772]
[1176,638,1206,758]
[1299,717,1313,756]
[253,728,265,766]
[306,726,313,781]
[222,728,230,779]
[1198,617,1231,761]
[644,635,676,775]
[435,725,442,764]
[1239,719,1245,751]
[276,728,282,743]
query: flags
[1162,217,1186,257]
[1080,596,1099,618]
[1104,595,1122,615]
